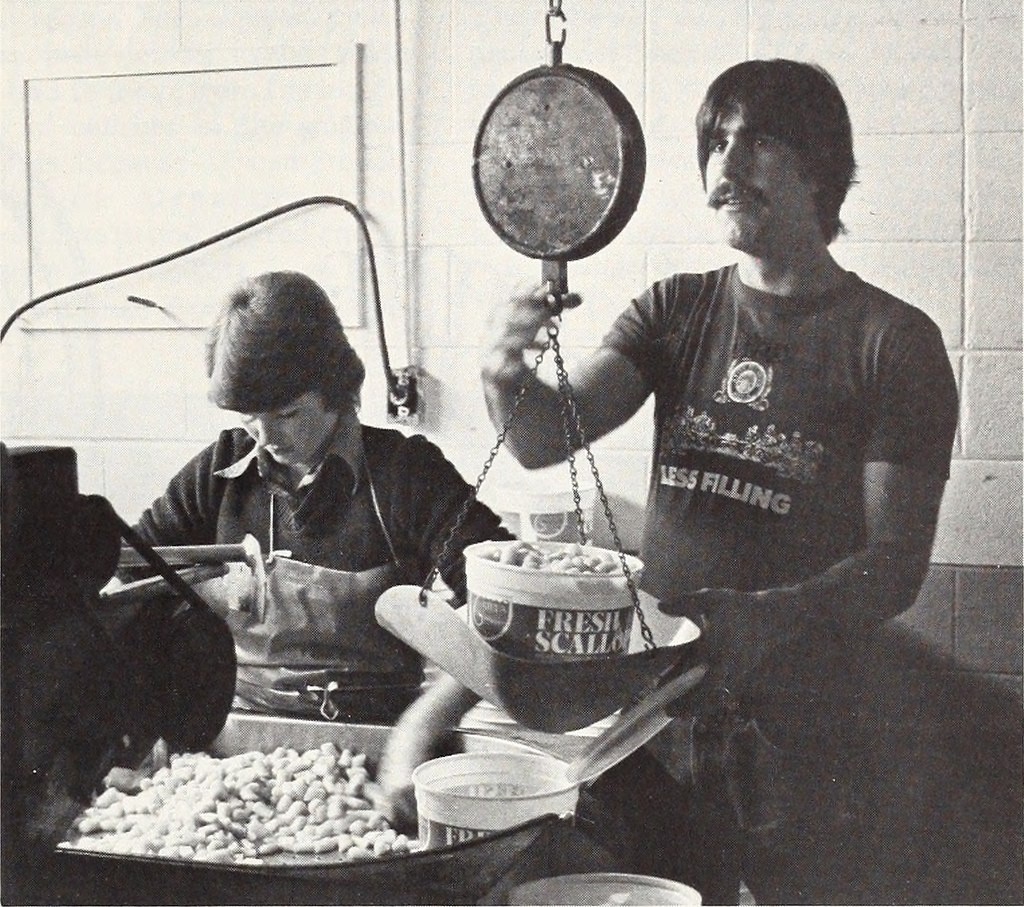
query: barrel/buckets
[510,871,701,904]
[412,752,582,856]
[460,539,645,661]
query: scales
[375,40,706,737]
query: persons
[483,59,957,658]
[110,272,517,834]
